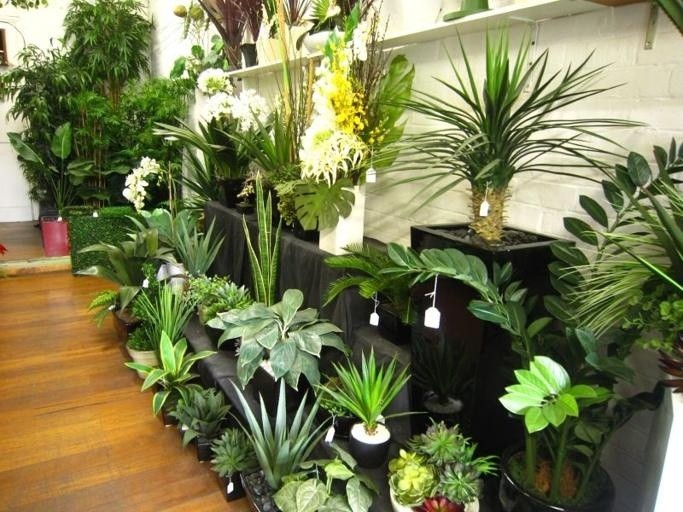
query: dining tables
[127,327,159,380]
[221,1,262,67]
[185,275,233,318]
[227,374,335,512]
[198,283,255,353]
[299,0,414,257]
[387,415,499,512]
[312,345,414,467]
[210,427,260,502]
[167,390,232,461]
[311,375,362,441]
[381,136,683,512]
[0,40,91,233]
[87,262,159,339]
[296,0,382,54]
[211,3,310,226]
[130,280,199,390]
[198,1,250,71]
[208,288,349,417]
[271,163,319,239]
[545,137,683,512]
[256,0,315,67]
[348,9,650,357]
[151,114,273,208]
[236,178,255,215]
[8,122,93,257]
[125,331,216,427]
